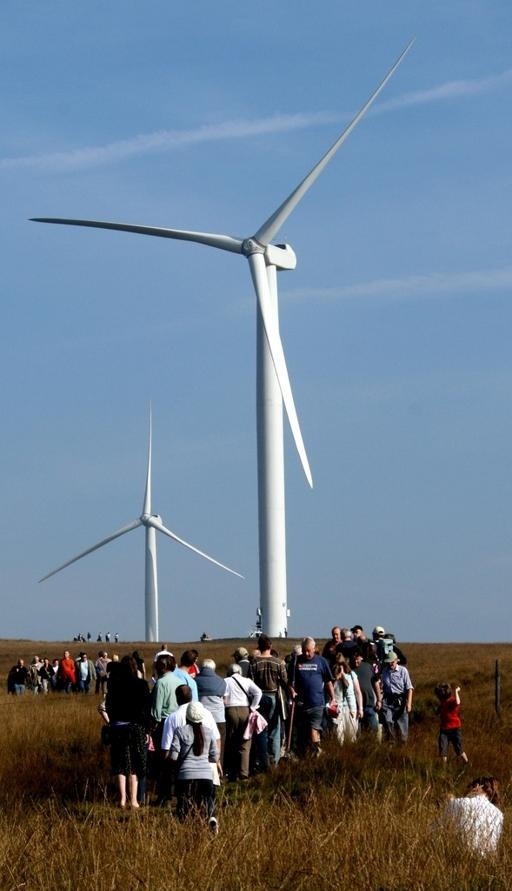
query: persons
[7,650,120,696]
[250,625,415,773]
[98,644,263,838]
[74,631,118,643]
[430,681,472,769]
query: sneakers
[208,816,219,836]
[117,803,126,810]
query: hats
[230,647,250,660]
[373,626,385,637]
[384,651,398,664]
[185,700,205,724]
